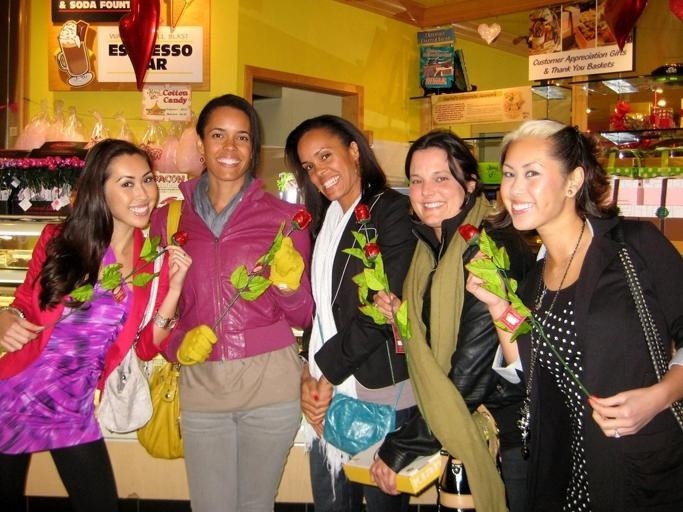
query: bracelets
[0,306,23,318]
[152,311,179,331]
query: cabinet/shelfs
[0,214,70,310]
[407,68,683,146]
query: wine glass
[56,36,93,87]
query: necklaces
[516,215,587,461]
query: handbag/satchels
[138,359,187,461]
[323,392,395,457]
[97,344,151,435]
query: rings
[614,429,619,438]
[373,304,377,307]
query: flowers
[176,207,313,370]
[0,156,86,218]
[458,223,592,401]
[341,202,432,436]
[0,229,189,356]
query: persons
[462,116,683,512]
[143,93,317,512]
[0,137,192,500]
[281,111,441,512]
[370,122,534,511]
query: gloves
[267,236,303,290]
[175,324,217,365]
